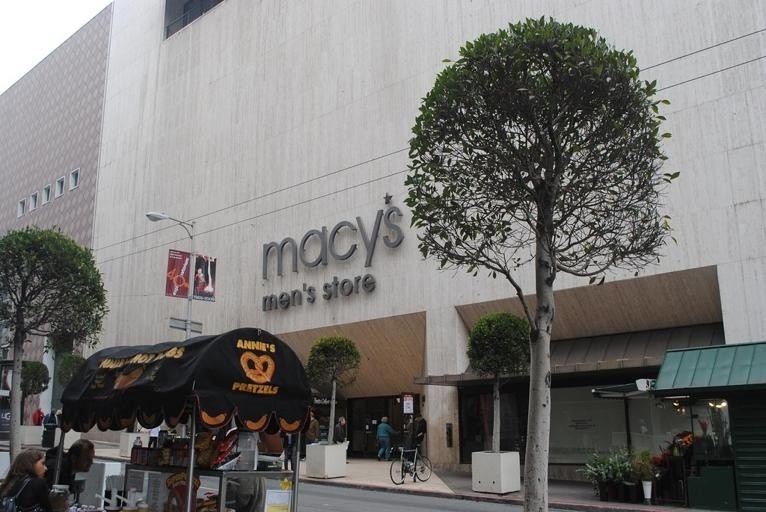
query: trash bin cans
[42,424,57,447]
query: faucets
[94,487,119,507]
[114,488,144,510]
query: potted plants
[467,310,530,493]
[305,334,360,479]
[19,354,86,448]
[576,449,659,502]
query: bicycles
[389,444,432,485]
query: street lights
[146,211,198,342]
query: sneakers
[377,455,391,461]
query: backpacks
[0,478,40,512]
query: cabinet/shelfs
[122,464,294,512]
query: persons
[31,408,44,425]
[0,448,52,511]
[409,413,427,461]
[280,429,297,470]
[299,412,319,460]
[45,438,94,511]
[43,408,56,425]
[377,416,400,460]
[333,417,349,464]
[147,424,160,448]
